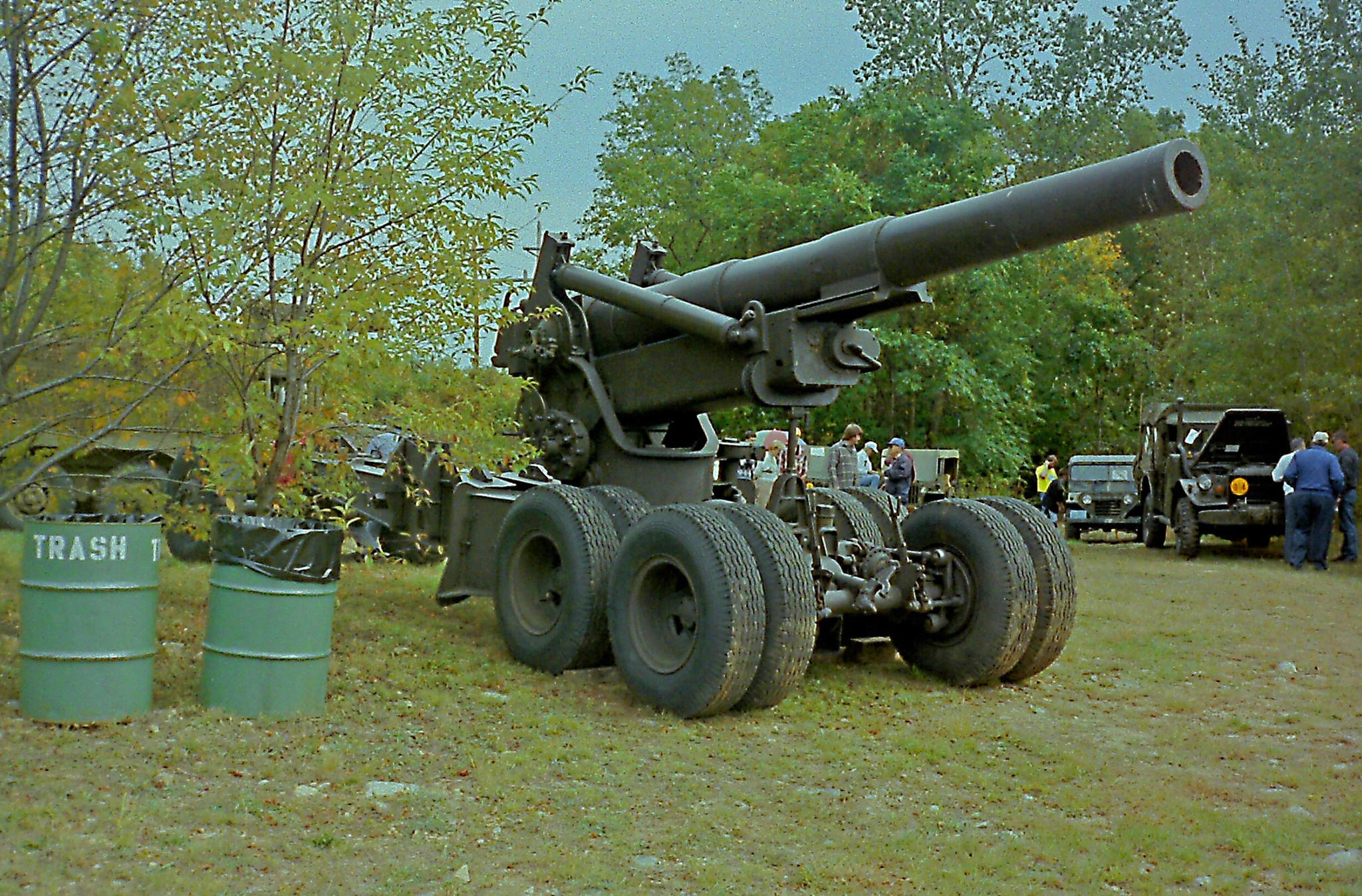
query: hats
[886,438,904,447]
[865,441,878,453]
[1312,431,1329,443]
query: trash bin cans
[198,513,344,719]
[20,520,162,722]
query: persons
[1035,454,1058,528]
[828,423,863,490]
[1271,437,1307,566]
[881,437,916,508]
[1040,467,1069,528]
[857,441,880,488]
[738,426,809,503]
[1282,431,1345,572]
[1332,433,1360,563]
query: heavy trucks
[1,419,220,532]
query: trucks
[1130,396,1307,560]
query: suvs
[1060,453,1142,540]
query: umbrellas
[745,428,807,449]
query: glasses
[889,445,892,449]
